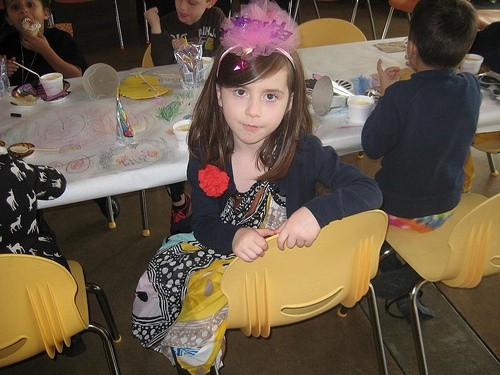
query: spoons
[10,145,59,153]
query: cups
[39,72,64,97]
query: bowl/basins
[202,57,214,80]
[347,95,375,123]
[173,119,191,141]
[460,54,484,75]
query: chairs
[0,0,500,375]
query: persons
[0,0,120,221]
[143,0,226,227]
[0,140,87,359]
[128,0,385,375]
[469,19,500,78]
[361,0,482,323]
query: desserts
[21,17,41,36]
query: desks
[0,35,500,209]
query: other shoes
[94,196,120,219]
[397,294,434,322]
[63,333,86,356]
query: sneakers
[170,194,191,235]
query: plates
[12,79,71,103]
